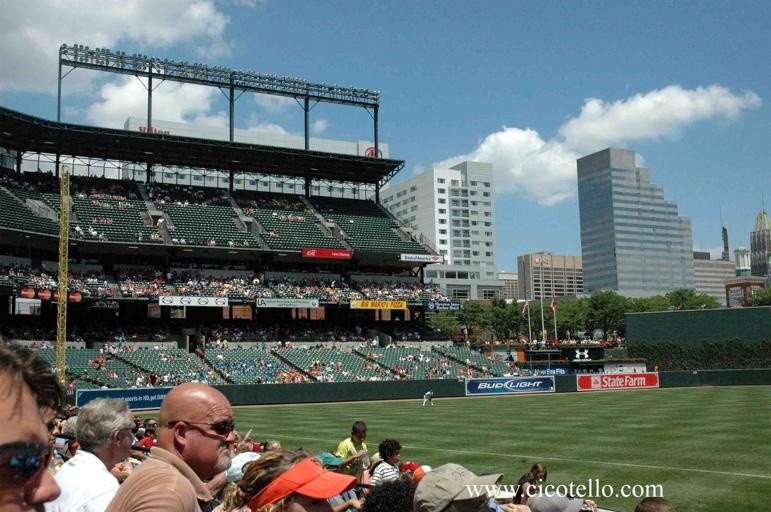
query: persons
[0,166,675,512]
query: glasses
[124,427,138,433]
[476,496,495,511]
[168,420,235,434]
[0,444,52,476]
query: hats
[246,458,357,511]
[526,493,583,512]
[316,452,343,469]
[227,452,261,482]
[400,460,504,512]
